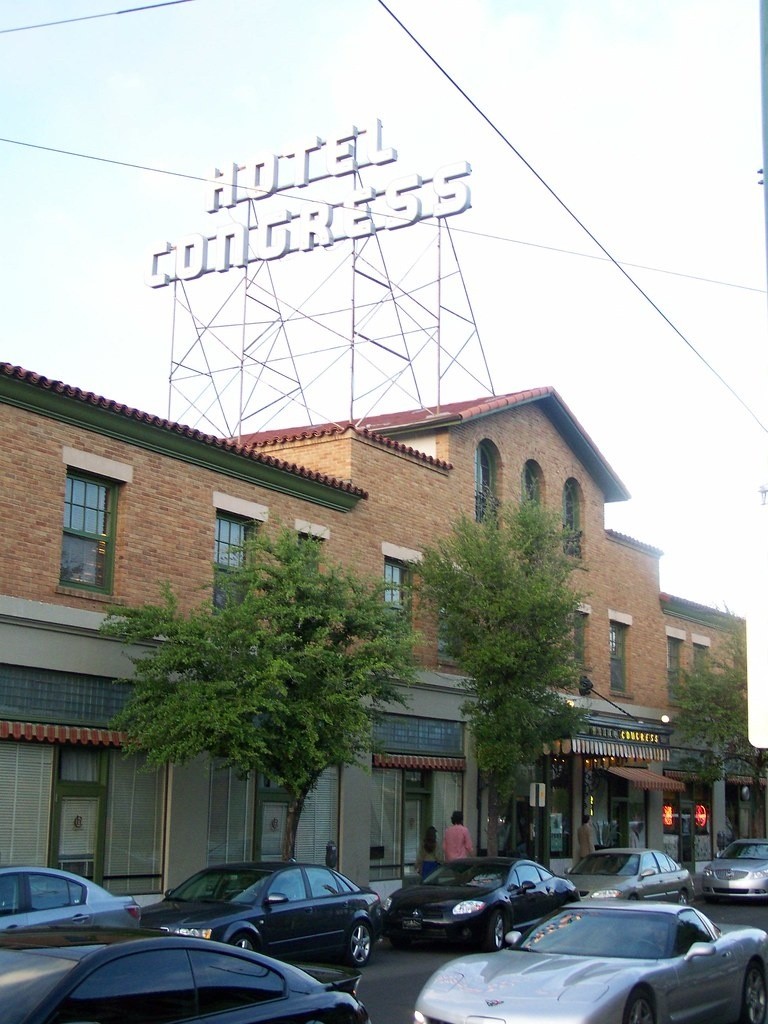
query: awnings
[603,766,686,793]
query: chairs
[226,877,257,901]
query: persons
[442,810,474,860]
[576,815,596,861]
[419,826,438,885]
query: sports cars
[412,898,768,1024]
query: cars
[636,814,733,866]
[700,838,768,903]
[0,925,373,1024]
[560,848,697,906]
[140,860,381,968]
[379,856,581,952]
[0,866,142,931]
